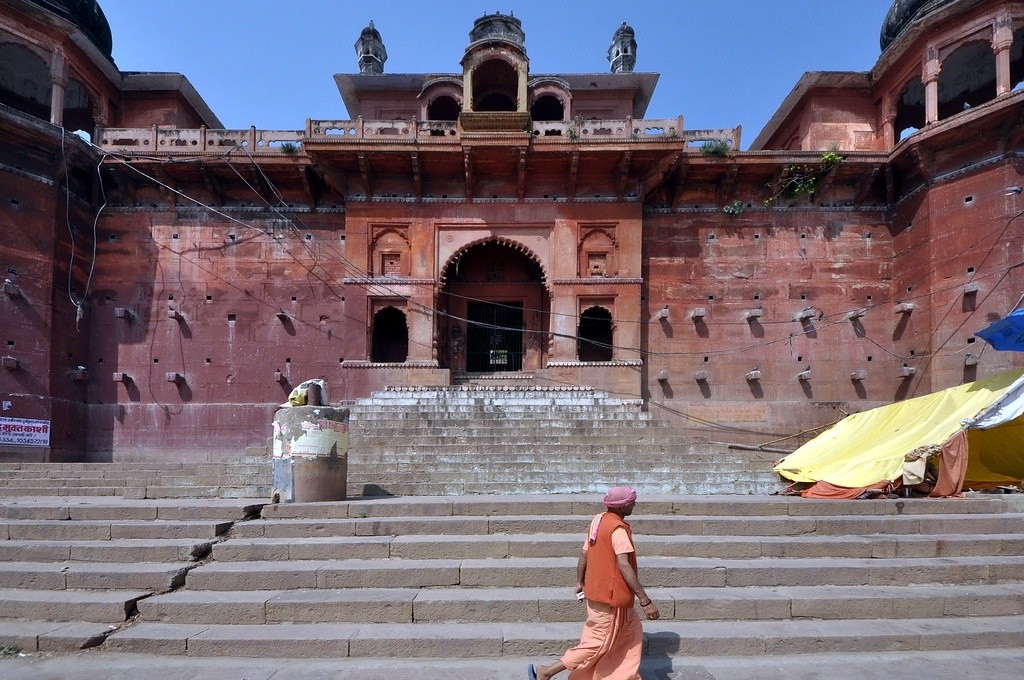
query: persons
[528,487,660,680]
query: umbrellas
[972,306,1024,353]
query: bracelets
[640,598,651,607]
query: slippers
[528,664,537,680]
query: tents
[772,367,1024,499]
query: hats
[603,486,637,507]
